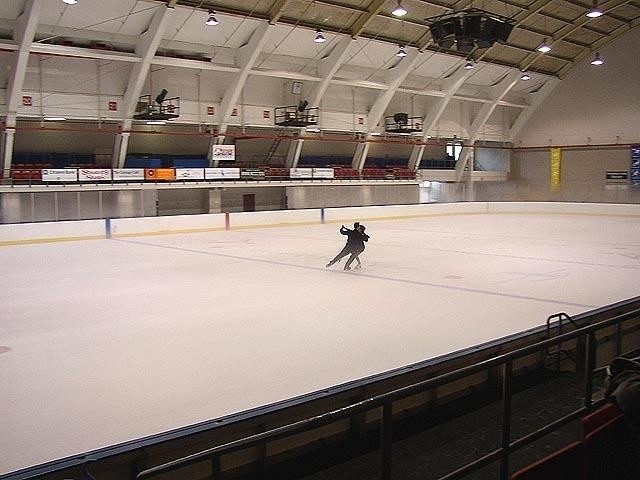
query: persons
[341,224,370,269]
[324,222,366,270]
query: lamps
[205,0,408,58]
[537,0,605,65]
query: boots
[355,262,362,267]
[344,266,352,271]
